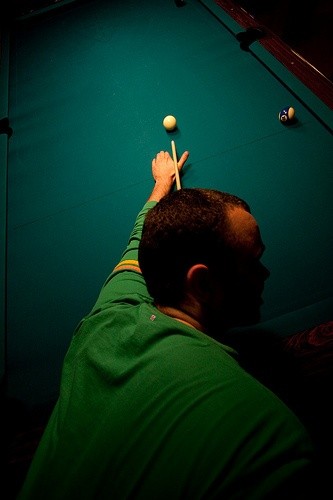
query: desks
[0,0,332,466]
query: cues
[171,140,181,190]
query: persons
[19,149,326,500]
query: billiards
[163,114,176,130]
[279,106,294,120]
